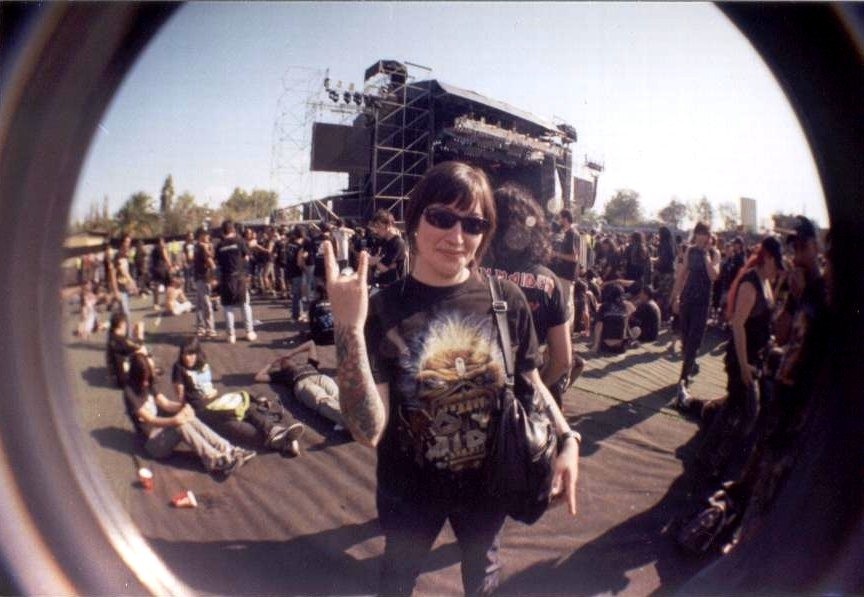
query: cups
[138,468,153,489]
[172,490,197,507]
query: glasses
[423,206,492,234]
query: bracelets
[560,431,582,452]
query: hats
[786,214,816,242]
[761,236,783,272]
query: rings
[341,272,350,277]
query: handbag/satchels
[487,275,556,526]
[204,390,250,421]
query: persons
[660,212,831,555]
[69,201,674,481]
[324,164,582,597]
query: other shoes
[673,379,689,408]
[269,423,304,450]
[232,446,255,460]
[286,439,301,456]
[226,331,235,343]
[195,328,205,334]
[222,454,242,476]
[244,330,257,341]
[207,328,214,340]
